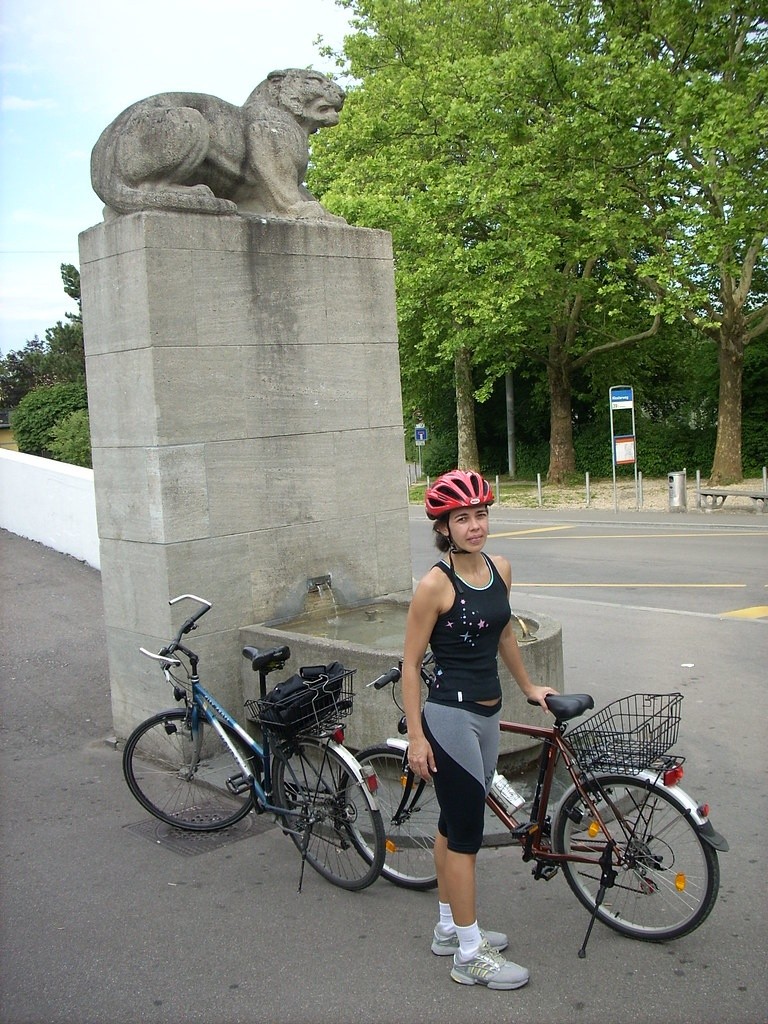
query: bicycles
[119,594,390,895]
[340,652,730,960]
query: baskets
[244,666,357,741]
[562,691,683,777]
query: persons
[400,468,561,990]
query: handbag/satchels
[263,661,344,736]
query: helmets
[425,469,494,519]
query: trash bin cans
[668,471,687,507]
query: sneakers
[430,920,508,956]
[451,938,529,990]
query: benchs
[694,489,768,513]
